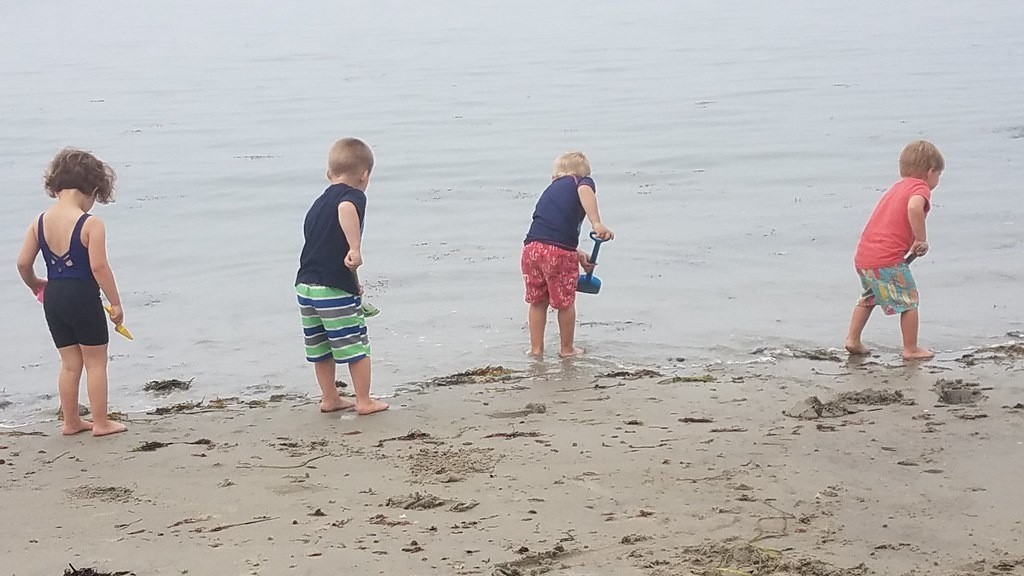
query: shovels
[103,304,134,341]
[575,229,610,297]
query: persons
[295,139,390,415]
[18,148,129,436]
[520,152,613,357]
[846,141,944,358]
